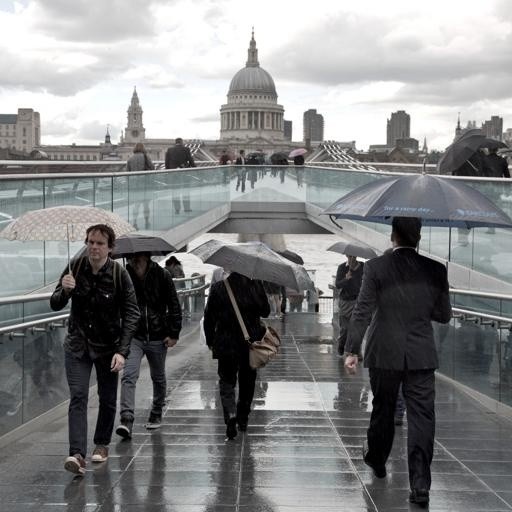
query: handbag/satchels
[246,325,283,369]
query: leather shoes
[362,439,387,478]
[408,487,430,505]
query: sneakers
[115,424,132,442]
[91,444,109,462]
[184,209,193,212]
[174,211,180,214]
[226,415,238,440]
[237,410,247,431]
[146,422,162,429]
[393,415,403,426]
[63,453,87,475]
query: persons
[164,138,198,214]
[437,148,512,247]
[204,265,320,442]
[336,217,452,506]
[222,144,311,195]
[124,145,156,229]
[50,224,190,473]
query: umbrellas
[437,128,508,173]
[318,154,512,268]
[0,203,139,280]
